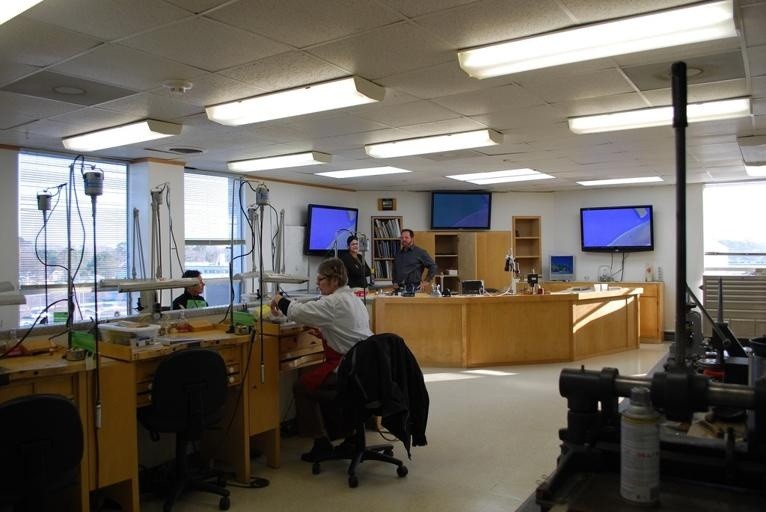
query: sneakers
[301,438,335,462]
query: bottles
[127,309,189,349]
[375,284,407,296]
[431,283,442,297]
[0,328,20,353]
[621,386,661,507]
[233,322,254,335]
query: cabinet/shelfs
[411,227,459,288]
[248,334,277,435]
[513,280,667,344]
[458,230,513,292]
[81,357,134,492]
[369,213,403,282]
[511,213,542,279]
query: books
[374,219,400,279]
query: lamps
[232,204,270,282]
[458,1,740,85]
[561,92,757,138]
[0,280,28,306]
[96,205,166,292]
[255,206,309,286]
[57,117,184,154]
[205,71,388,131]
[363,126,508,160]
[115,199,204,296]
[225,146,333,175]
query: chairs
[303,330,431,489]
[462,278,486,294]
[0,391,86,512]
[139,346,237,511]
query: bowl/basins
[65,349,86,361]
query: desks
[90,321,252,512]
[261,321,329,471]
[1,340,92,511]
[359,285,647,367]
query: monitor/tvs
[431,191,491,230]
[304,204,358,256]
[580,205,654,252]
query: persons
[270,257,375,462]
[392,229,437,292]
[172,270,208,310]
[341,236,372,288]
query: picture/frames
[376,198,396,211]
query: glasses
[317,277,327,284]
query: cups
[594,284,600,292]
[534,283,545,295]
[746,337,766,433]
[602,284,608,291]
